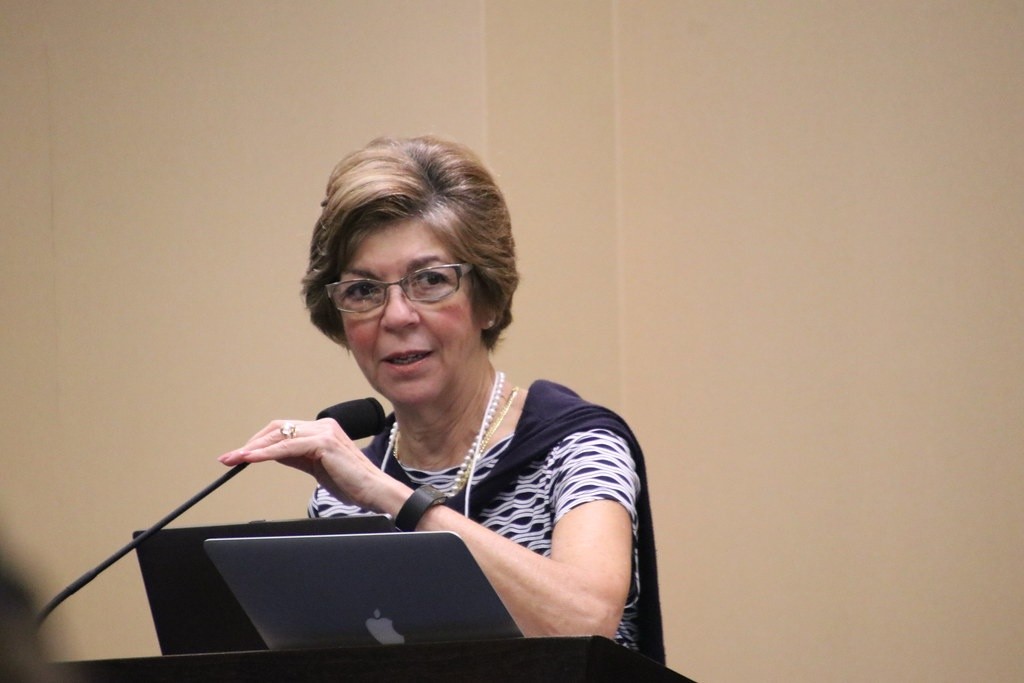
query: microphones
[34,396,386,635]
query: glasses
[325,263,475,314]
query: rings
[281,422,296,439]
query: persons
[217,135,668,666]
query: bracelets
[394,484,449,533]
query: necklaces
[380,368,519,516]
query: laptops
[131,513,397,655]
[203,530,524,651]
